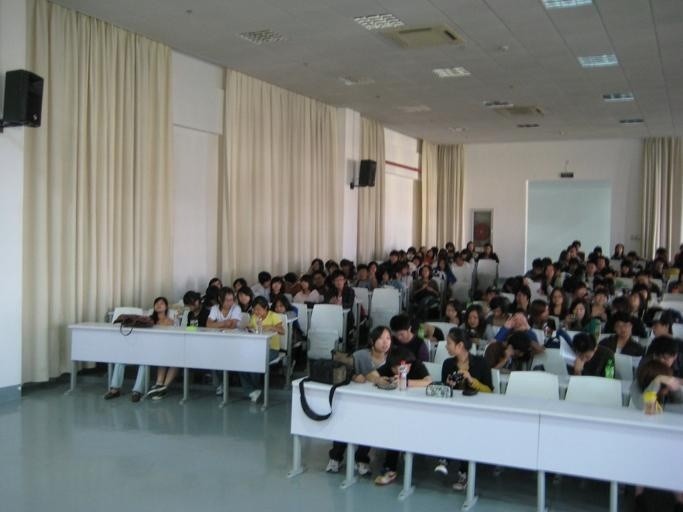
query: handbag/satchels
[309,351,354,385]
[113,313,154,336]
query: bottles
[398,360,407,391]
[604,360,614,379]
[172,313,178,329]
[256,315,263,334]
[417,323,424,339]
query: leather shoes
[131,391,140,402]
[103,388,120,399]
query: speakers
[358,159,376,186]
[4,69,44,128]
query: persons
[623,358,683,512]
[104,219,683,402]
[365,347,432,486]
[325,325,392,477]
[432,327,494,490]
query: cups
[189,319,197,329]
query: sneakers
[325,457,344,473]
[355,461,373,475]
[374,467,400,485]
[249,388,262,404]
[149,383,169,400]
[452,471,467,490]
[433,459,448,479]
[215,382,222,395]
